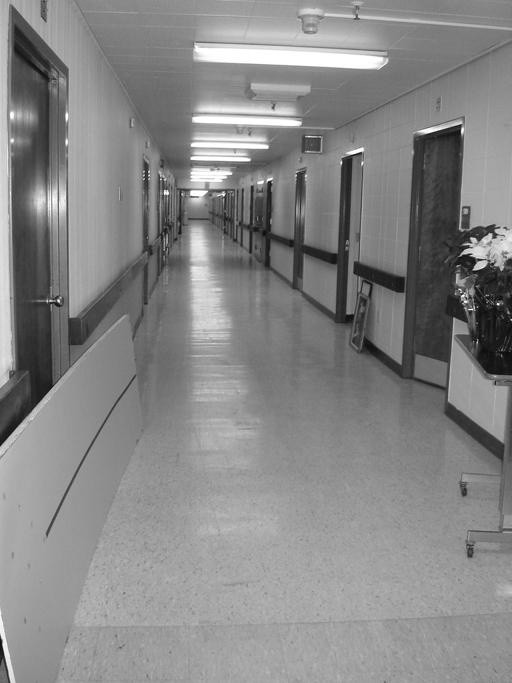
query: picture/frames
[348,293,369,352]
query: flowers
[445,224,512,354]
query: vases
[461,295,510,356]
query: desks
[454,332,511,560]
